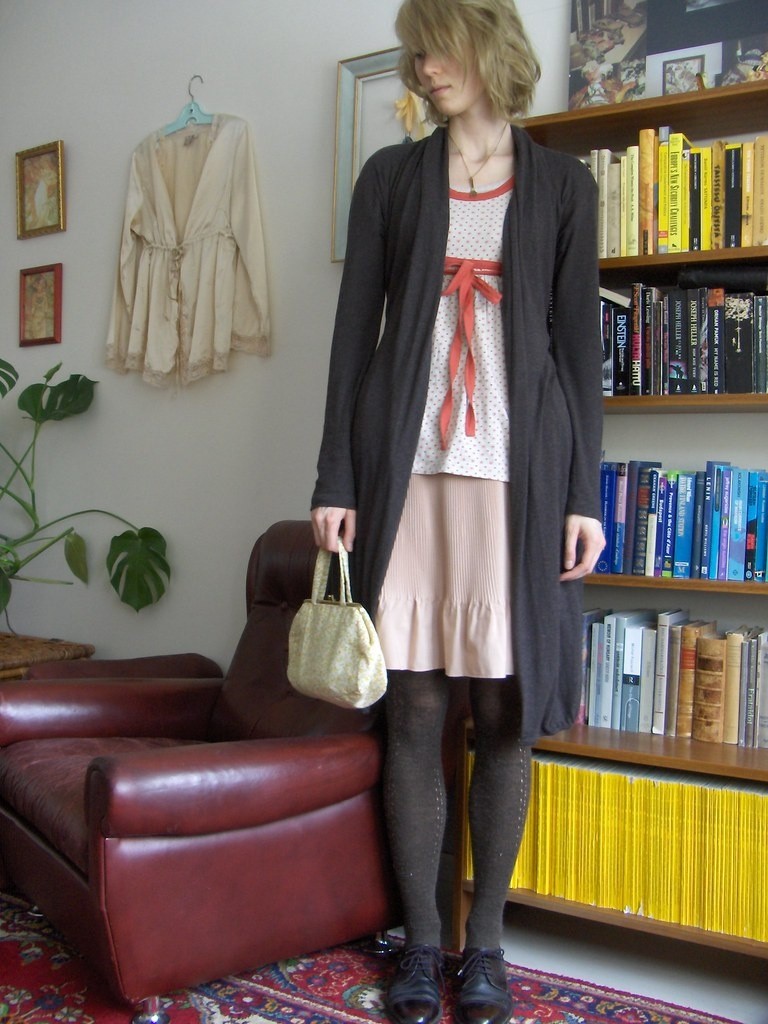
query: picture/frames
[15,141,65,243]
[331,45,437,262]
[19,263,61,345]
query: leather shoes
[385,943,448,1024]
[455,946,514,1024]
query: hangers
[153,72,214,141]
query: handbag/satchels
[286,535,389,711]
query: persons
[309,1,604,1024]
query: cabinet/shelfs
[444,83,764,966]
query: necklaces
[445,122,508,196]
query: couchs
[0,512,404,1024]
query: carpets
[0,887,745,1023]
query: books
[590,125,768,260]
[593,460,768,583]
[599,280,768,396]
[581,612,768,752]
[461,750,768,946]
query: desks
[0,632,95,682]
